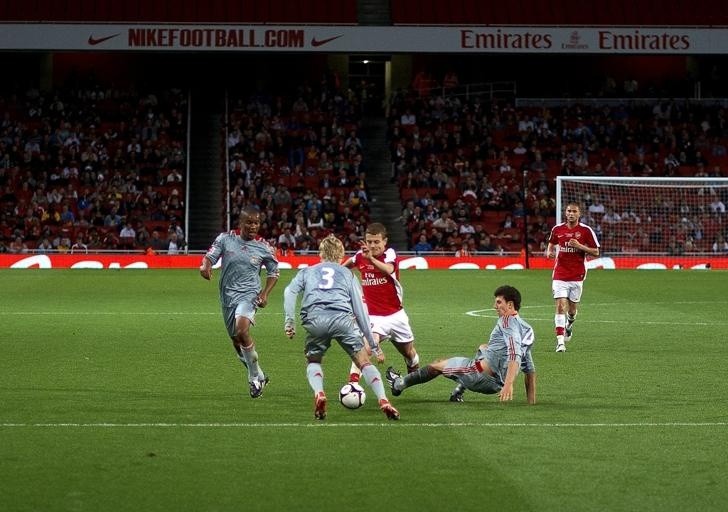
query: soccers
[339,384,365,409]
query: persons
[339,223,420,384]
[386,286,536,406]
[0,50,728,256]
[200,205,281,398]
[282,233,401,422]
[546,201,600,352]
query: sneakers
[378,398,400,420]
[314,391,327,420]
[450,390,464,402]
[386,367,402,396]
[556,343,566,352]
[564,323,572,342]
[248,372,270,398]
